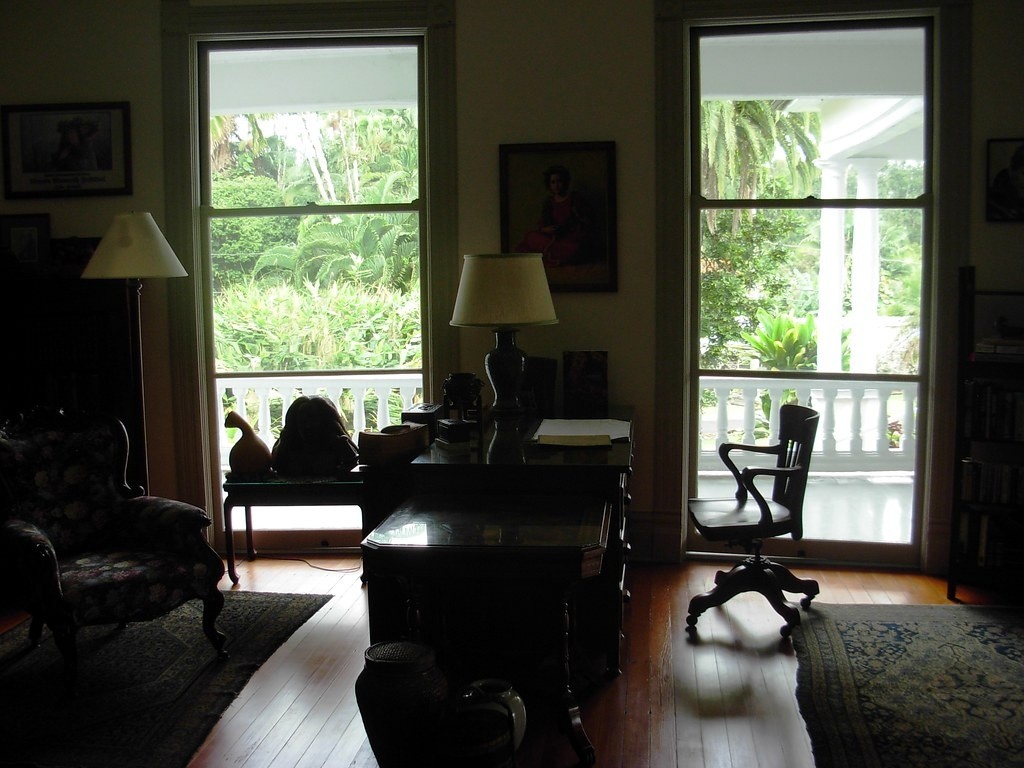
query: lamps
[449,254,559,417]
[81,213,189,496]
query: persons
[269,399,357,476]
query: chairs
[686,405,819,636]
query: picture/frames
[0,213,53,271]
[0,101,133,200]
[501,141,618,292]
[986,137,1024,224]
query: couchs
[0,399,230,697]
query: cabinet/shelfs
[946,265,1024,598]
[0,272,143,496]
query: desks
[361,494,614,767]
[411,404,636,676]
[223,473,378,585]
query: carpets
[0,590,331,768]
[790,601,1024,768]
